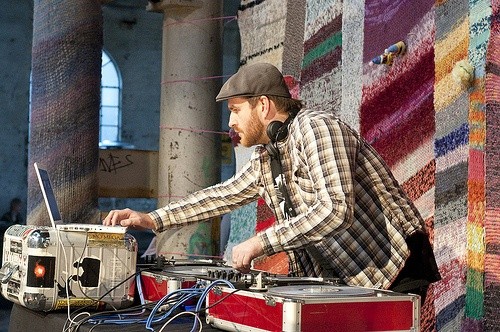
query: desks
[8,303,229,332]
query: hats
[214,62,292,103]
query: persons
[102,63,441,307]
[0,198,24,225]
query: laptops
[34,162,128,233]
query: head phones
[264,106,300,160]
